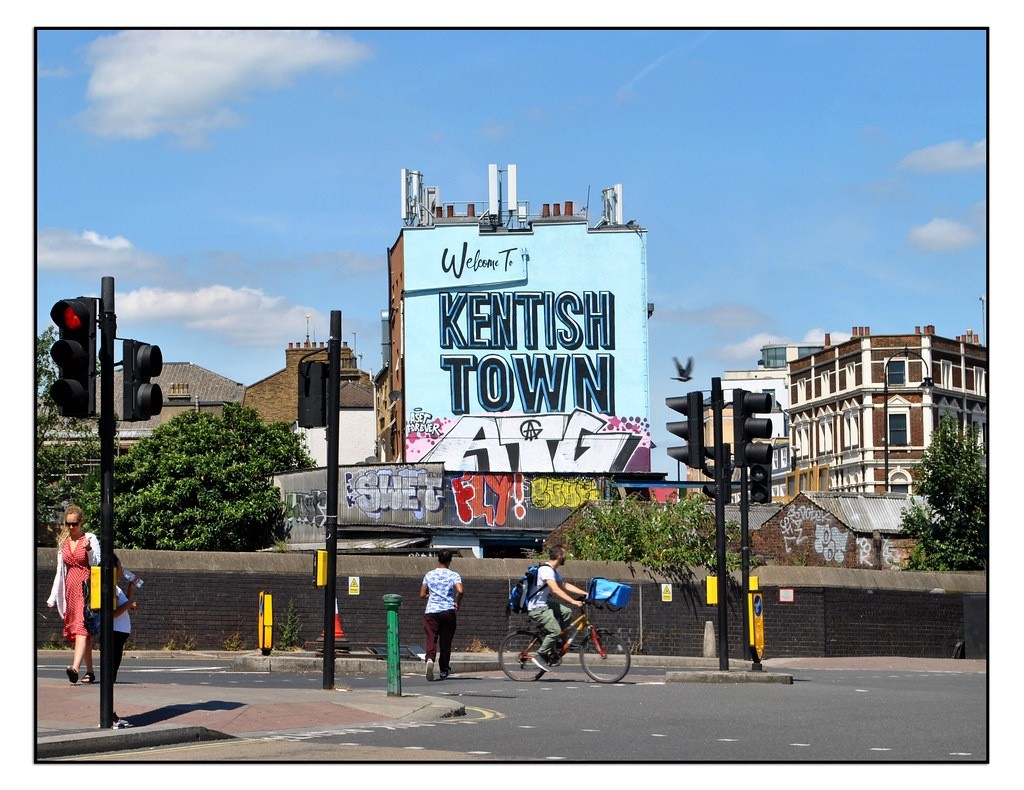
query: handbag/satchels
[590,576,632,612]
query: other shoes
[66,667,78,683]
[531,654,552,672]
[426,659,434,681]
[440,671,448,679]
[113,720,134,729]
[81,672,95,683]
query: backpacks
[506,564,557,617]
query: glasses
[65,522,79,526]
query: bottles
[122,570,144,588]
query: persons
[421,550,464,681]
[528,545,589,674]
[47,505,101,684]
[98,554,140,730]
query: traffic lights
[50,297,96,418]
[733,389,773,468]
[123,339,164,422]
[666,391,704,469]
[702,443,732,505]
[750,464,772,503]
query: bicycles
[498,594,631,684]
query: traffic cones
[316,598,349,642]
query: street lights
[884,351,938,492]
